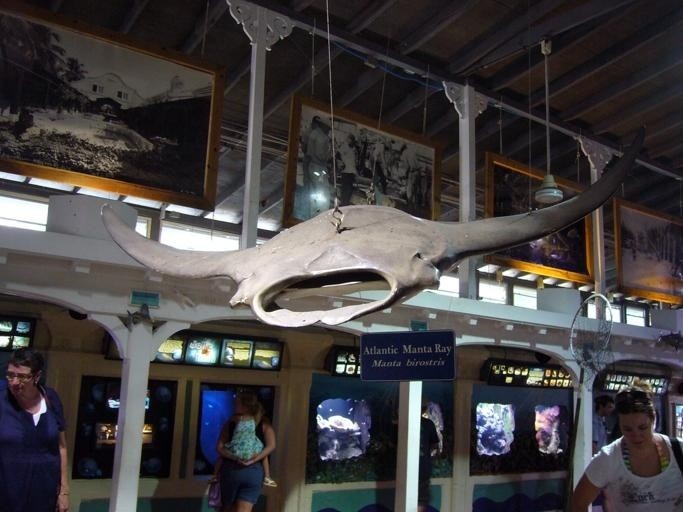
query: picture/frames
[0,0,226,212]
[484,150,595,286]
[614,196,683,305]
[280,93,442,228]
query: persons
[417,394,440,511]
[217,395,275,511]
[591,391,618,459]
[206,391,277,487]
[0,346,70,512]
[571,383,683,510]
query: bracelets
[57,491,71,496]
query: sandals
[263,477,277,488]
[208,477,217,484]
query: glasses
[6,374,28,383]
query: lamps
[534,38,565,204]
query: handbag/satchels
[208,474,222,508]
